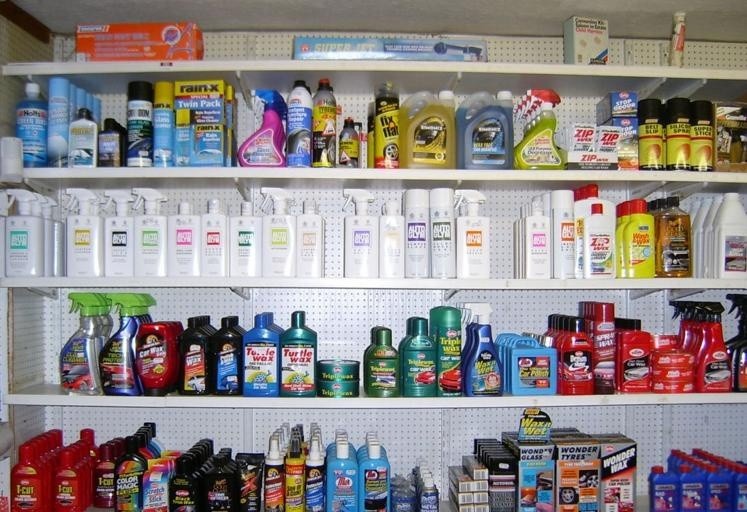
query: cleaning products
[2,185,746,280]
[58,292,746,399]
[7,407,747,512]
[0,71,746,171]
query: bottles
[171,423,234,511]
[648,447,745,512]
[546,300,656,395]
[513,181,747,281]
[287,77,400,166]
[171,195,261,280]
[637,96,713,171]
[295,199,328,279]
[134,308,317,396]
[17,76,172,167]
[379,188,456,277]
[669,9,689,65]
[326,419,391,511]
[9,423,166,511]
[364,306,465,395]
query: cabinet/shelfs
[1,1,747,512]
